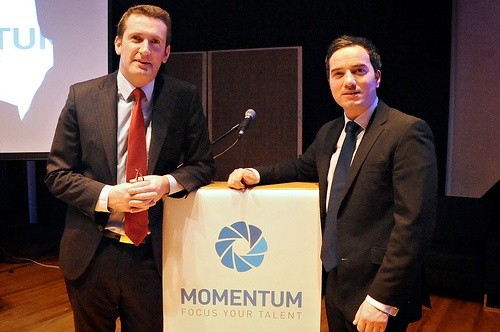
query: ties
[124,87,149,247]
[320,121,363,274]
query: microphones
[236,109,256,137]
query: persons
[44,5,216,332]
[227,34,438,332]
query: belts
[105,228,150,244]
